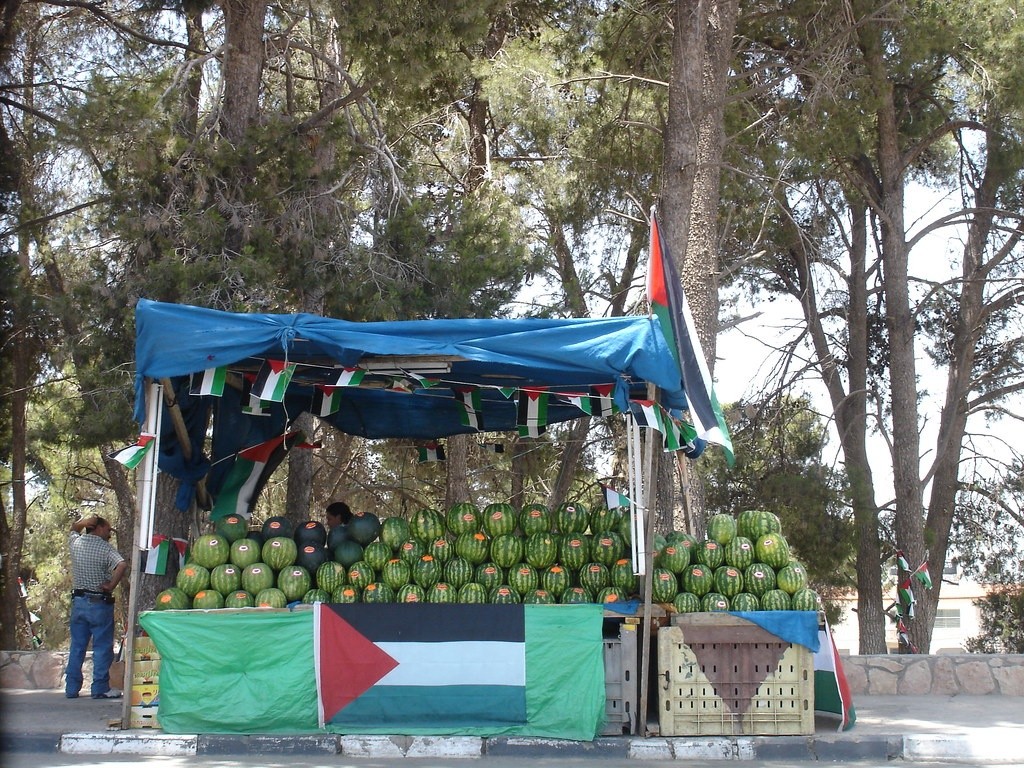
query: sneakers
[67,693,79,698]
[92,688,121,699]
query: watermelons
[649,510,821,614]
[154,501,638,610]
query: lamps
[333,362,452,375]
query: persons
[65,516,128,699]
[326,502,354,528]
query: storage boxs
[131,659,159,685]
[133,636,160,660]
[131,684,159,707]
[596,622,637,734]
[657,626,817,736]
[130,706,162,728]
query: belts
[76,592,106,600]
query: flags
[107,432,156,470]
[189,359,369,417]
[140,533,191,576]
[207,429,301,522]
[313,601,527,732]
[384,366,698,464]
[107,298,706,737]
[647,211,736,468]
[17,576,43,649]
[894,550,933,624]
[600,484,629,511]
